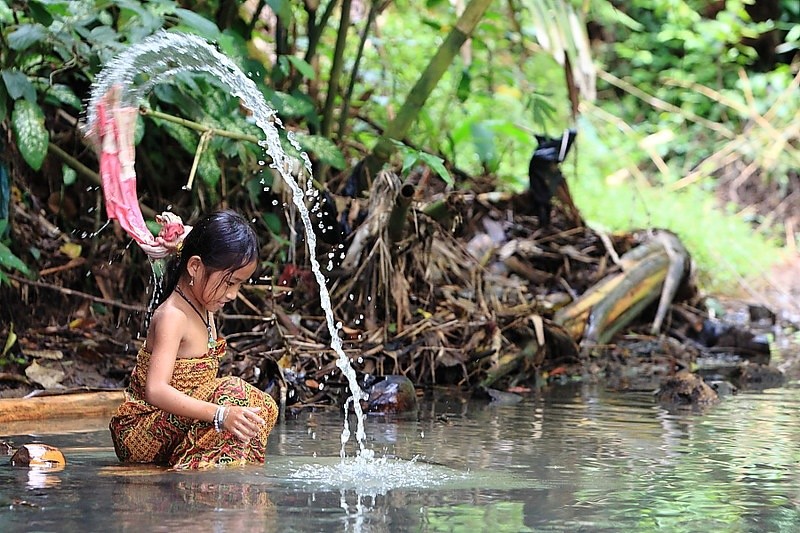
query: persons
[108,209,280,471]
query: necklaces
[172,284,218,348]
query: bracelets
[214,405,230,433]
